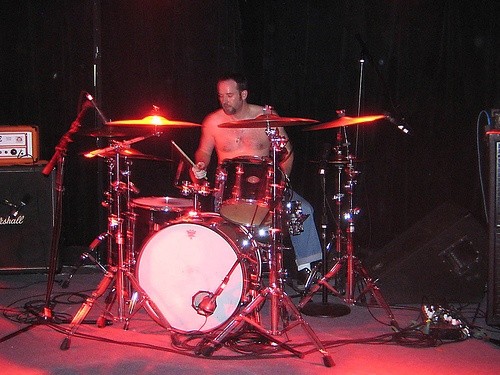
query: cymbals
[79,145,168,160]
[105,117,201,127]
[80,127,157,136]
[314,115,386,130]
[217,115,318,128]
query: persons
[192,73,323,285]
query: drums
[220,155,285,226]
[129,196,200,270]
[134,212,262,333]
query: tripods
[0,141,182,351]
[200,122,400,367]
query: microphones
[120,169,139,195]
[384,112,409,133]
[85,93,106,123]
[199,277,229,313]
[174,161,184,185]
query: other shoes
[297,269,322,290]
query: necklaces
[233,129,244,144]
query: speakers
[0,165,60,273]
[485,133,500,326]
